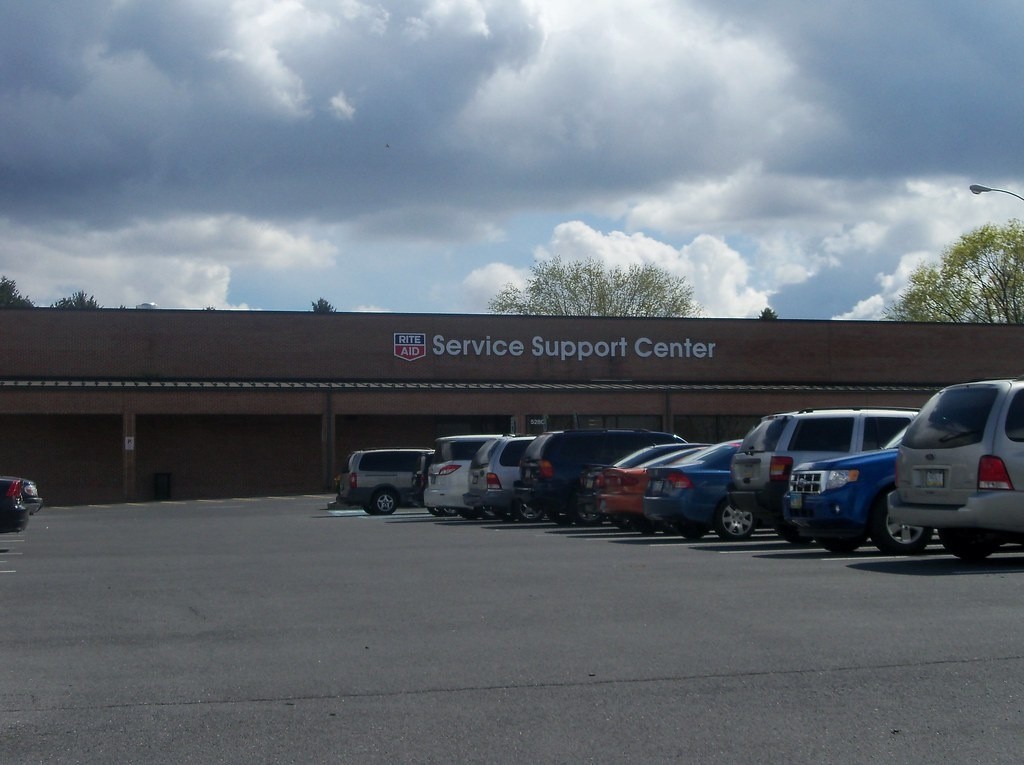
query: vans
[424,434,505,521]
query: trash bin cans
[152,473,172,500]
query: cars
[0,475,42,532]
[579,446,709,532]
[641,439,756,542]
[782,427,934,557]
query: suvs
[467,434,547,522]
[732,408,917,541]
[514,428,686,526]
[886,379,1022,555]
[341,446,435,515]
